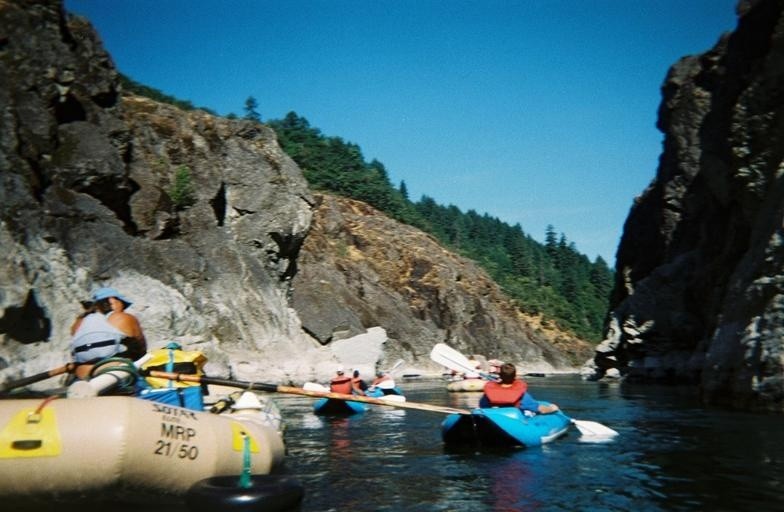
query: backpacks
[70,310,131,364]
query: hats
[90,288,134,310]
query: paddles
[303,381,331,394]
[430,343,619,437]
[374,358,405,383]
[139,367,471,416]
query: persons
[375,360,384,378]
[463,355,482,379]
[330,367,395,397]
[480,359,558,415]
[69,287,147,397]
